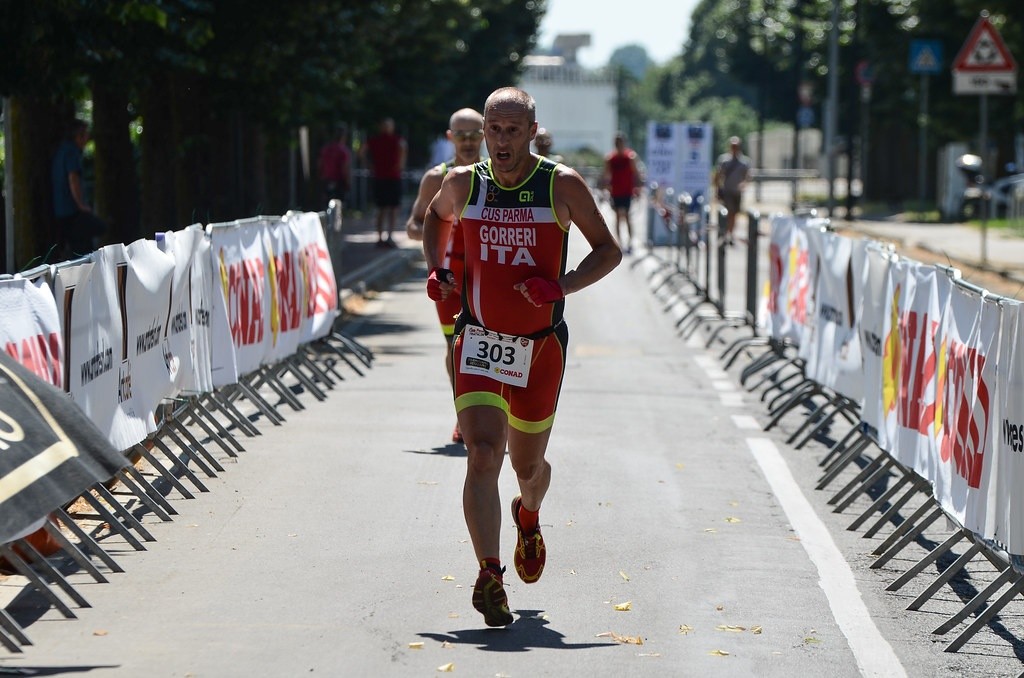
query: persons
[318,122,352,208]
[50,118,105,260]
[407,106,484,442]
[358,117,408,248]
[533,135,555,156]
[604,135,641,237]
[422,86,622,625]
[712,136,753,245]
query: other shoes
[452,420,464,442]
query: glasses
[450,129,484,142]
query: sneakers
[512,495,546,583]
[471,569,514,626]
